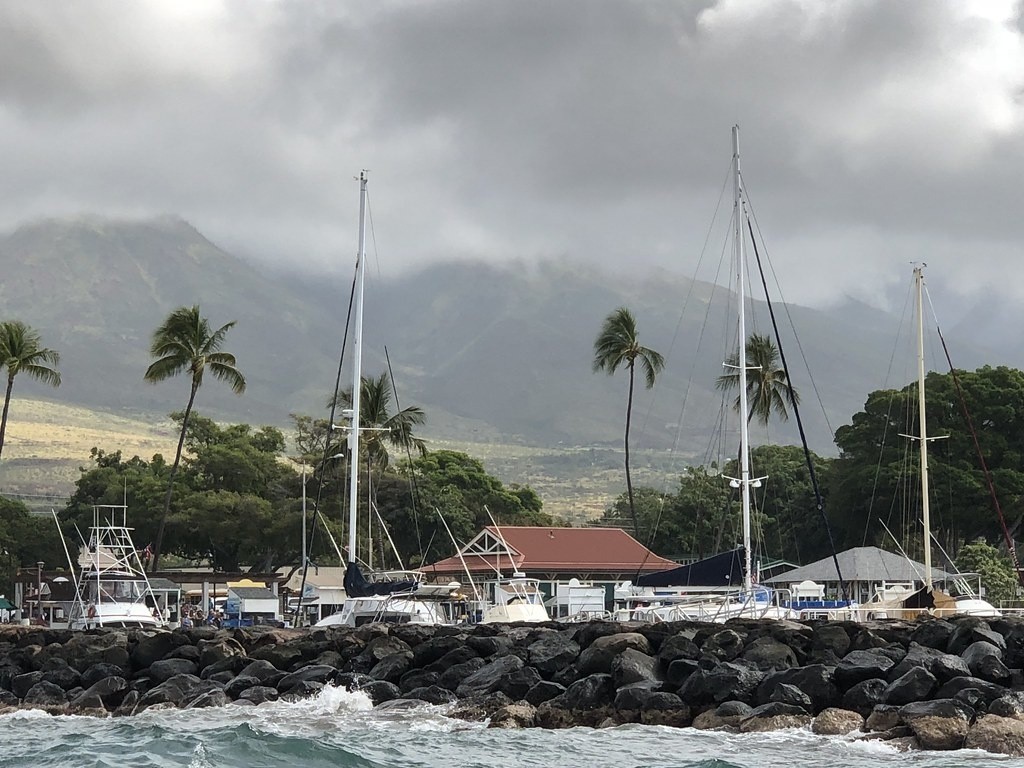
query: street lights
[37,562,45,616]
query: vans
[285,597,315,614]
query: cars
[196,597,228,613]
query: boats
[70,504,165,628]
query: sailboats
[283,121,1024,628]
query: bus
[185,590,229,608]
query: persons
[182,603,222,628]
